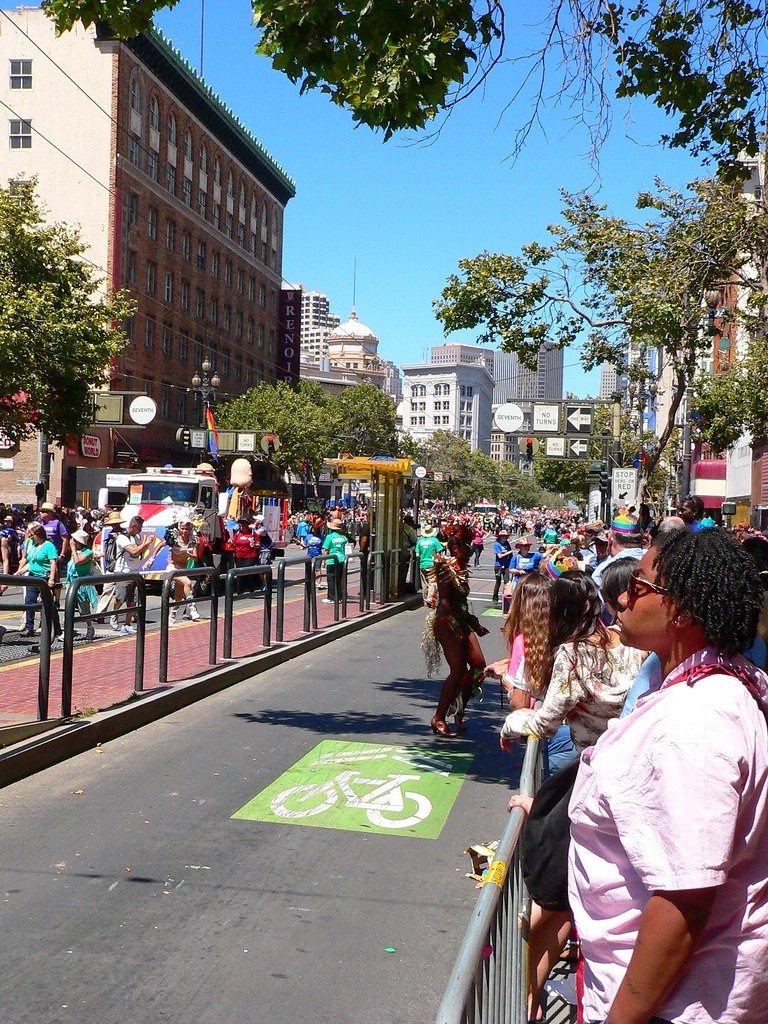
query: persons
[0,501,273,642]
[280,497,768,1024]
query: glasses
[626,572,671,606]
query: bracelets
[50,577,54,581]
[186,551,189,555]
[60,554,65,558]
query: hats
[253,515,265,523]
[4,516,14,522]
[496,530,509,537]
[515,537,533,549]
[39,502,56,513]
[104,512,127,525]
[71,530,89,545]
[327,519,342,530]
[592,529,613,542]
[237,516,250,524]
[421,525,436,537]
[403,515,414,520]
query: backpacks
[106,534,131,572]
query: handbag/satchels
[89,559,103,586]
[343,541,354,563]
[518,733,601,912]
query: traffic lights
[181,431,190,446]
[267,435,274,455]
[526,438,533,457]
[599,472,608,492]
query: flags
[206,403,220,464]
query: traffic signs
[566,406,592,433]
[568,438,589,457]
[612,468,637,507]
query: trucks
[90,465,290,599]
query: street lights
[681,287,721,498]
[626,363,658,485]
[192,355,220,464]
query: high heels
[454,712,466,731]
[430,716,458,738]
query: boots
[187,594,200,620]
[167,600,179,627]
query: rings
[482,629,484,632]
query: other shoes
[17,613,136,641]
[322,599,342,604]
